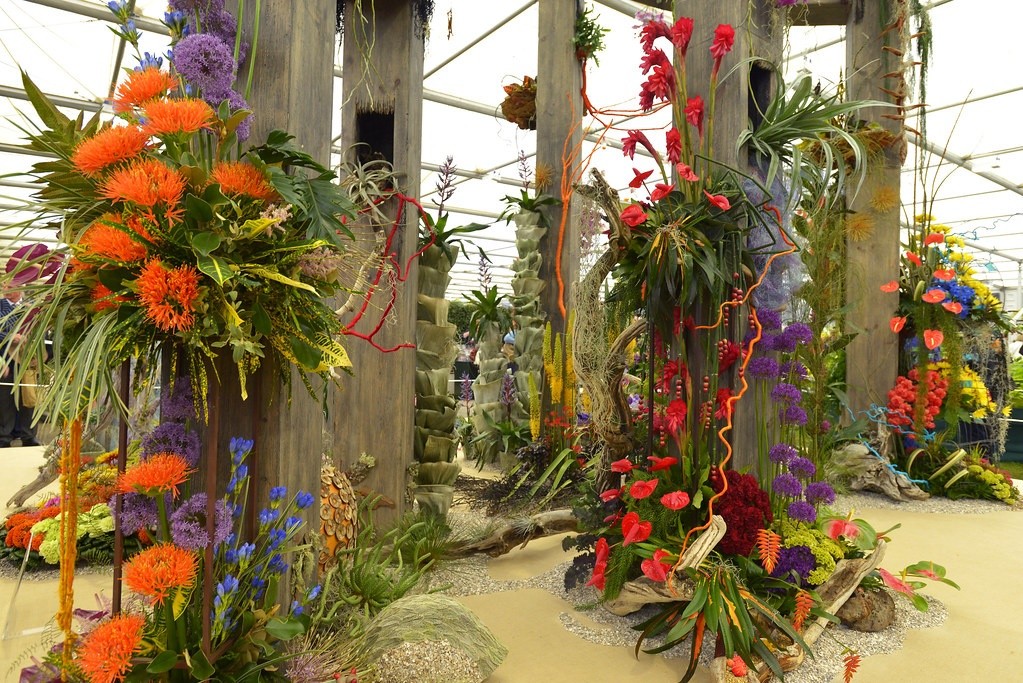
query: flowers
[0,1,1023,683]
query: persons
[0,279,44,448]
